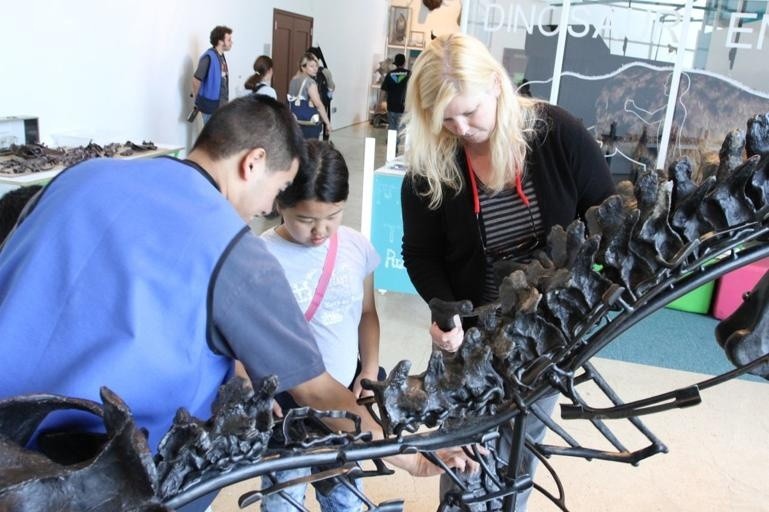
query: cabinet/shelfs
[385,5,427,66]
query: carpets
[583,303,769,384]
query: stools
[592,241,769,321]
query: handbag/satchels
[286,75,329,127]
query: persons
[285,52,332,141]
[251,139,381,512]
[305,46,336,141]
[0,92,492,512]
[402,35,631,512]
[191,26,235,128]
[378,52,411,156]
[243,55,278,98]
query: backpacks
[312,66,333,106]
